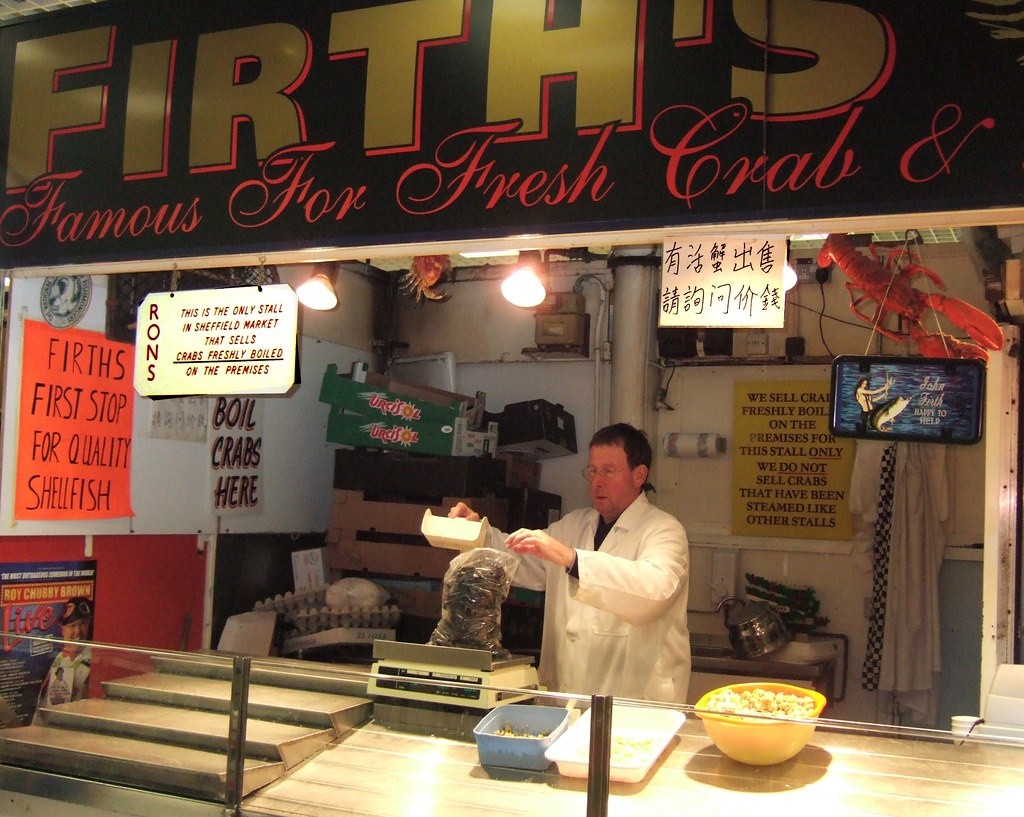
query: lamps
[296,264,338,311]
[784,240,798,293]
[501,250,546,307]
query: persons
[50,666,70,705]
[31,599,93,723]
[444,423,692,707]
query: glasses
[582,466,630,479]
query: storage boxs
[216,610,287,659]
[318,359,577,618]
[472,705,569,770]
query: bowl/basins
[474,705,570,770]
[695,683,827,765]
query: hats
[60,597,92,625]
[55,668,64,675]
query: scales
[365,637,540,710]
